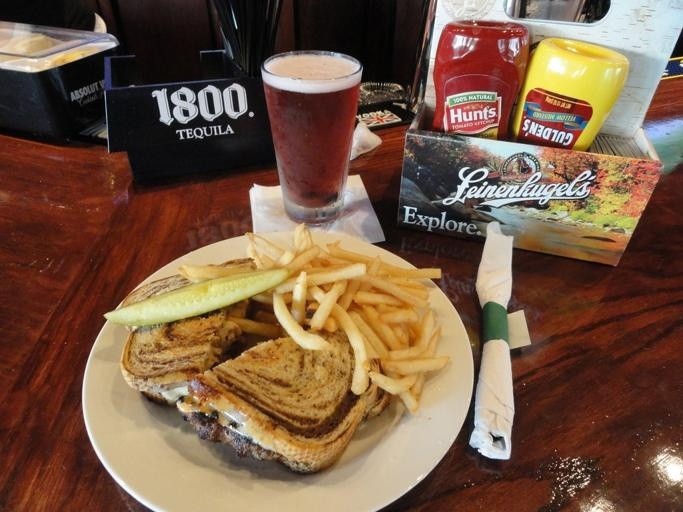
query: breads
[121,256,392,476]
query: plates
[82,231,476,512]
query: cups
[263,48,363,227]
[432,21,629,149]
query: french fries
[176,221,450,412]
